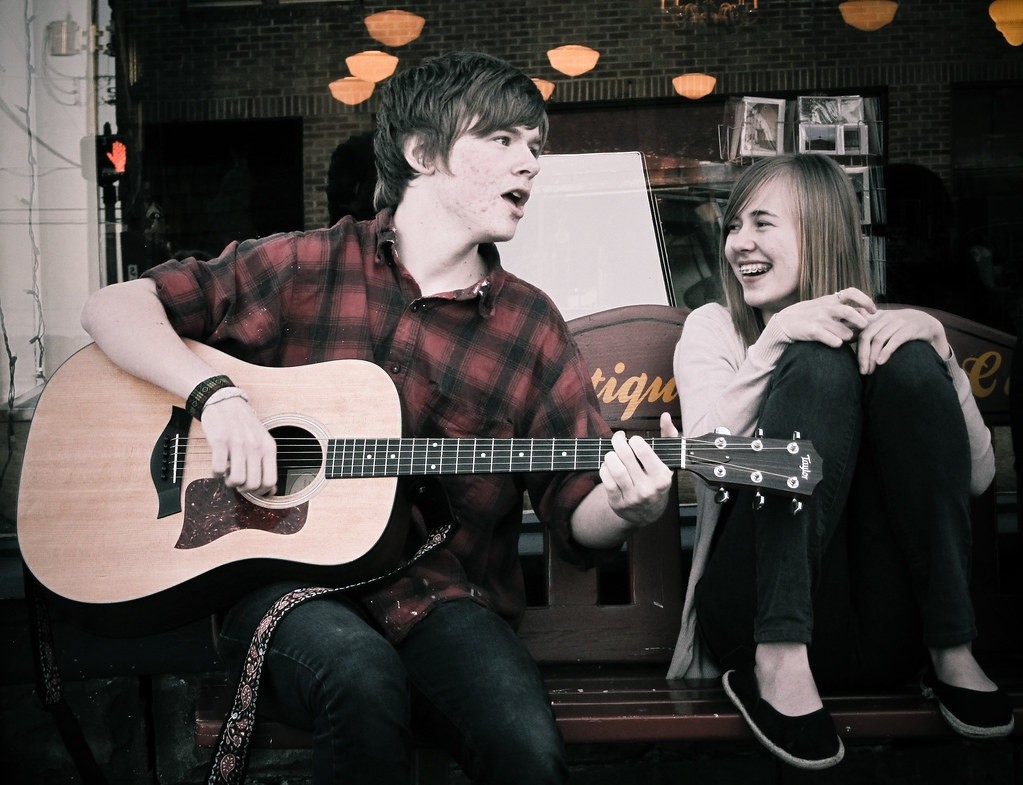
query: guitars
[14,336,822,642]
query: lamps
[658,0,758,39]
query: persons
[667,153,1014,769]
[81,50,679,785]
[811,100,822,124]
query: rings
[835,292,842,301]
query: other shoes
[924,647,1016,738]
[723,663,846,768]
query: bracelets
[187,375,250,421]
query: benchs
[145,304,1023,785]
